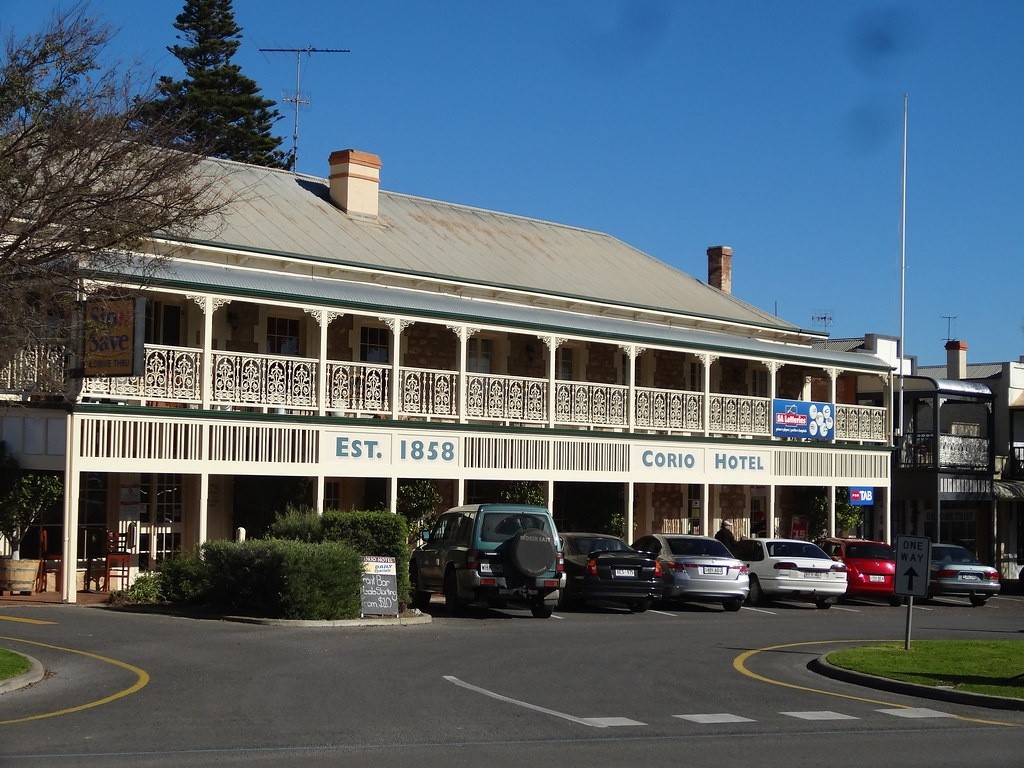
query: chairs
[103,528,131,592]
[38,530,62,592]
[84,553,108,591]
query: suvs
[815,537,900,607]
[409,503,567,618]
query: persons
[715,521,737,556]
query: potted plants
[0,441,65,591]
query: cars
[630,534,750,612]
[559,533,664,614]
[930,543,1001,606]
[735,538,848,610]
